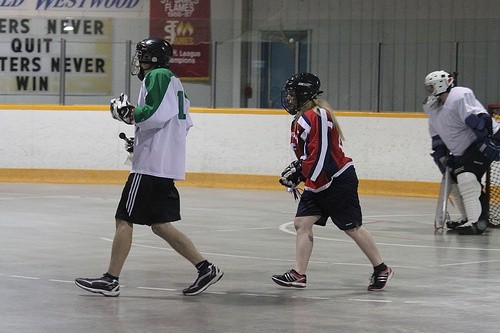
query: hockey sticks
[119,132,134,166]
[434,153,449,229]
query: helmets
[136,39,173,68]
[282,73,322,94]
[423,71,454,95]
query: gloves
[109,92,135,125]
[279,160,307,189]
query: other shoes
[446,219,467,230]
[454,222,484,234]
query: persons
[481,108,500,228]
[422,70,500,236]
[271,72,394,292]
[75,38,224,297]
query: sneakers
[367,266,394,291]
[75,276,120,297]
[182,264,224,295]
[271,269,307,288]
